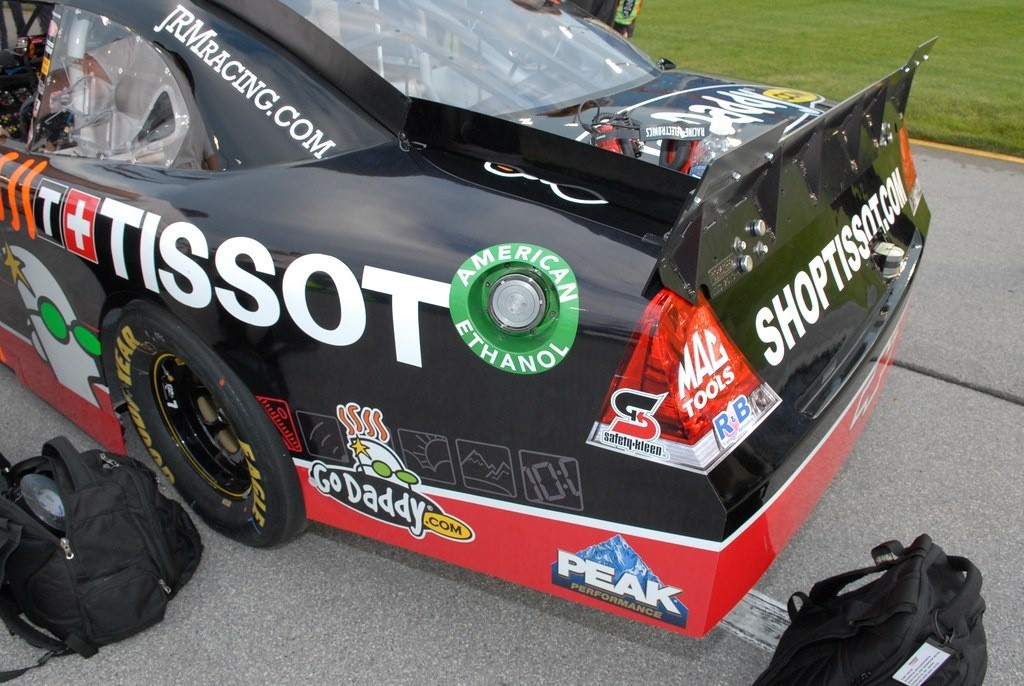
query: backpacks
[750,533,987,686]
[0,436,203,682]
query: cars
[1,0,934,640]
